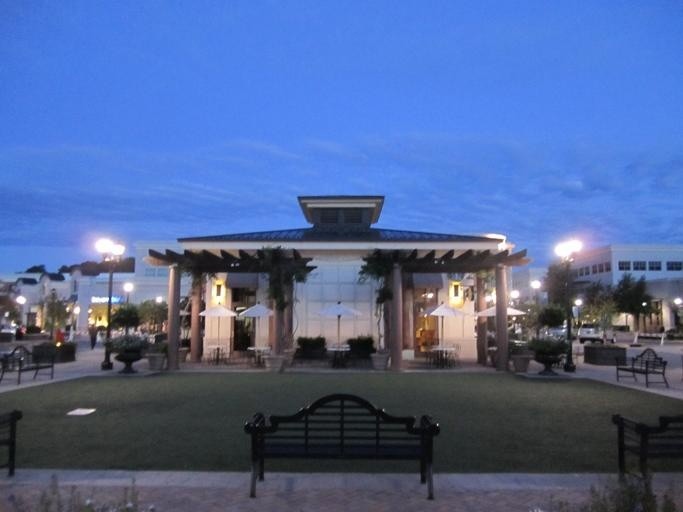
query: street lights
[124,282,133,334]
[93,239,124,371]
[554,238,581,372]
[47,288,56,339]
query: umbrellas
[308,300,363,344]
[238,300,275,347]
[196,301,237,345]
[472,302,527,318]
[417,299,468,346]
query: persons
[87,323,98,351]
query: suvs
[578,321,618,343]
[548,324,577,342]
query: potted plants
[529,336,569,376]
[108,334,148,374]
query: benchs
[1,346,56,385]
[615,347,670,389]
[611,412,683,491]
[244,392,441,500]
